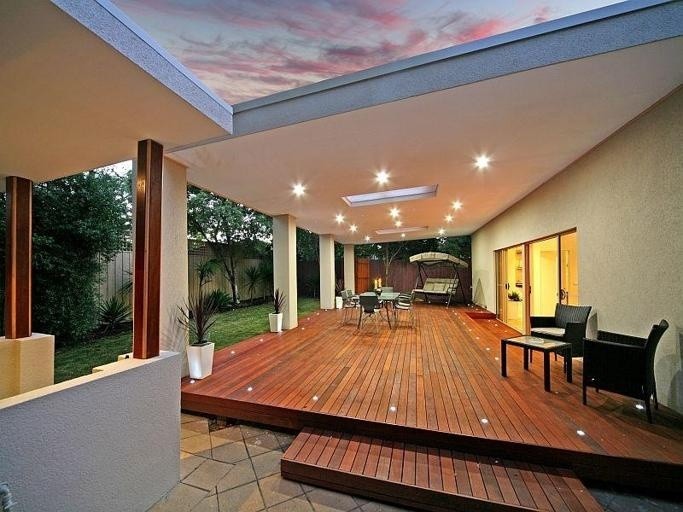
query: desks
[500,333,574,391]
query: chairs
[526,300,669,424]
[339,285,416,331]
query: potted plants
[175,288,228,380]
[266,288,287,332]
